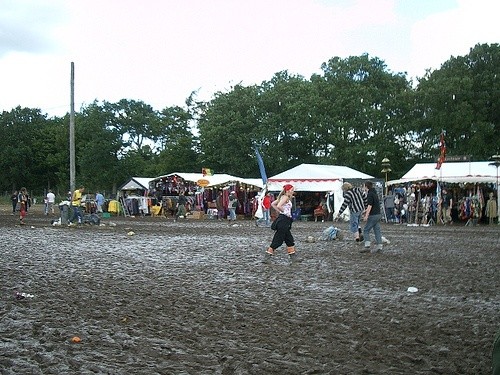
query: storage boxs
[193,212,214,220]
[301,215,312,222]
[102,213,110,217]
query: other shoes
[255,220,259,227]
[265,225,270,228]
[354,237,360,243]
[358,246,371,254]
[373,248,384,255]
[358,225,363,233]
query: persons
[360,181,383,253]
[11,186,452,228]
[332,182,368,242]
[260,185,303,264]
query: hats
[282,184,293,192]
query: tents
[119,161,500,223]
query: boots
[288,251,304,264]
[260,250,275,264]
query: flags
[202,168,214,177]
[434,133,445,170]
[255,148,268,184]
